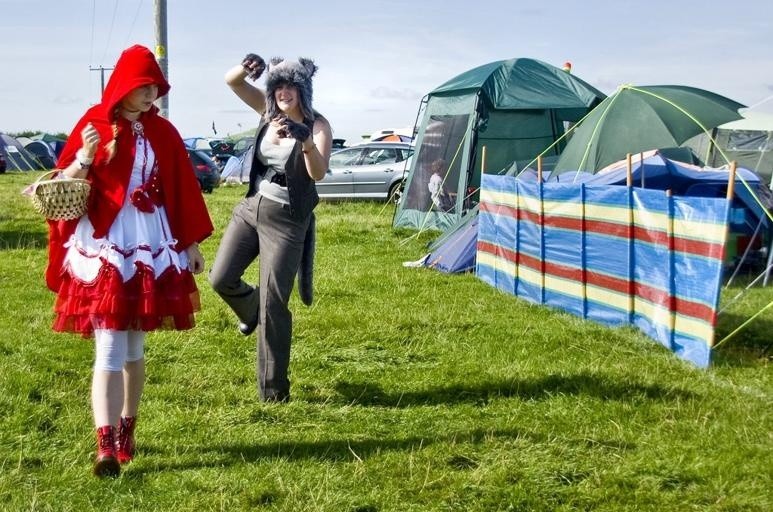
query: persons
[427,158,453,212]
[208,54,332,404]
[43,44,212,479]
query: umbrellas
[549,84,749,176]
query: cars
[187,149,220,194]
[315,141,415,206]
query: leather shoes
[237,284,259,337]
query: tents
[548,156,772,282]
[392,57,611,236]
[0,130,65,171]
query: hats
[266,54,317,122]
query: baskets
[27,168,90,221]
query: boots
[117,413,136,464]
[90,425,119,475]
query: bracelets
[72,157,89,170]
[74,150,93,165]
[302,145,316,155]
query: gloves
[241,53,265,79]
[276,114,311,142]
